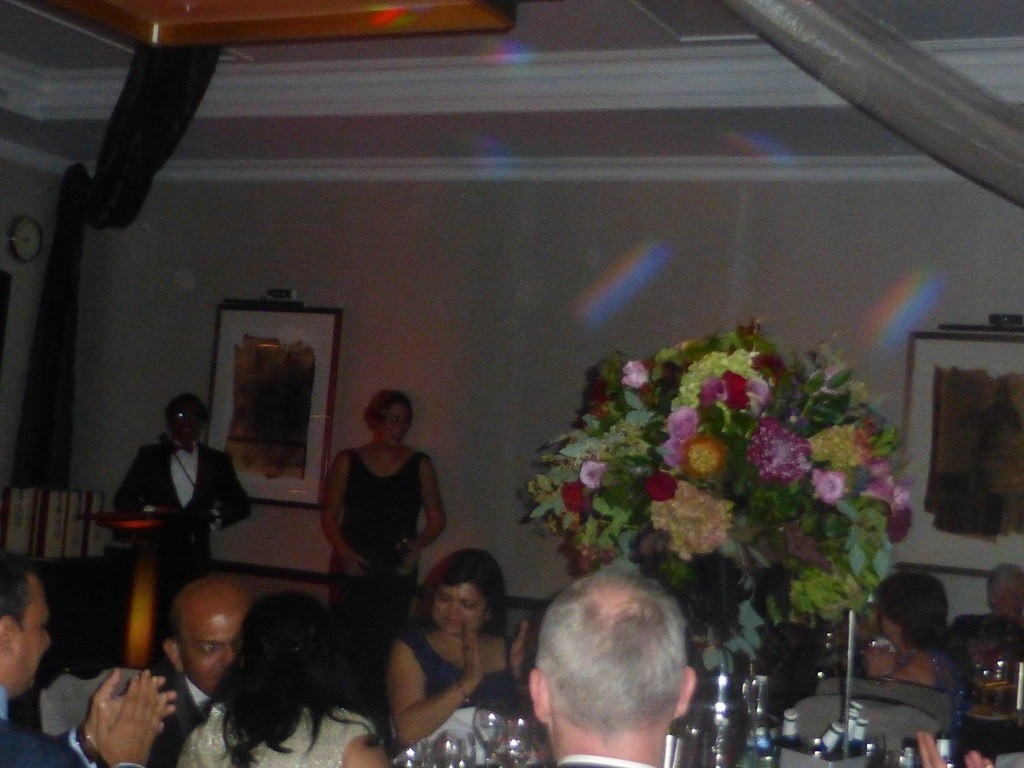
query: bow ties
[180,442,193,454]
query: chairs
[791,696,937,754]
[816,678,951,737]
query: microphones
[157,431,215,512]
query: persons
[839,563,1024,768]
[528,563,696,768]
[0,390,555,768]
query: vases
[672,650,750,768]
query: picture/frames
[899,331,1024,578]
[204,299,341,508]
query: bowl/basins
[138,497,173,517]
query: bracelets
[456,681,470,704]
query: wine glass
[473,699,503,768]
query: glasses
[391,415,411,424]
[176,412,203,423]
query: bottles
[663,663,805,768]
[816,632,881,680]
[975,655,1007,682]
[808,700,956,768]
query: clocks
[7,213,43,262]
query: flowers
[515,315,913,634]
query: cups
[982,670,991,681]
[392,729,488,768]
[495,711,549,768]
